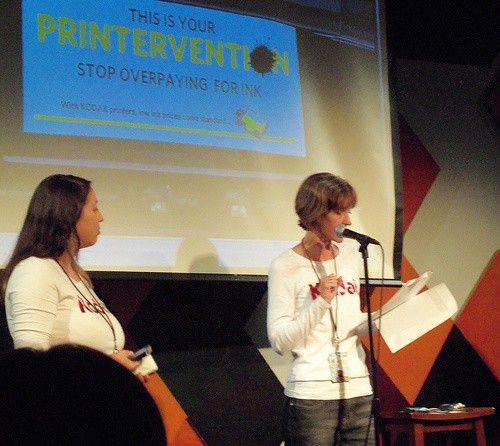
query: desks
[378,406,496,446]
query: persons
[264,172,380,446]
[0,173,151,446]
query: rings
[329,279,334,283]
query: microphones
[132,344,154,361]
[335,225,379,245]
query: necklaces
[52,257,120,356]
